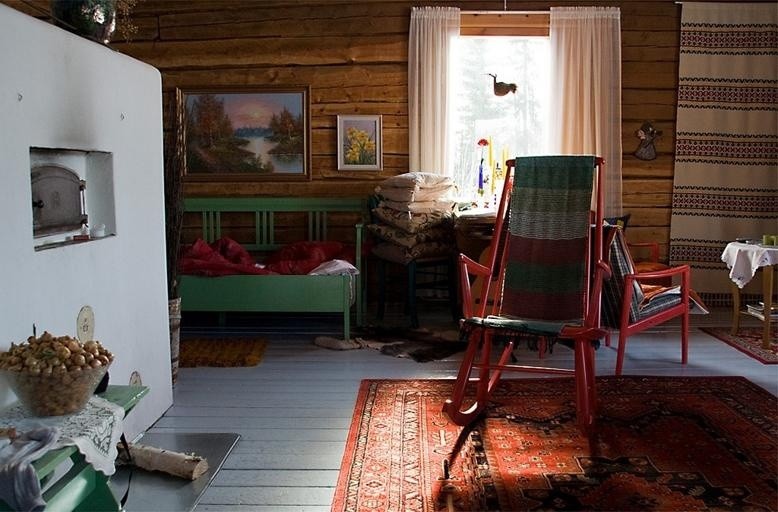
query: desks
[459,217,493,260]
[0,386,150,512]
[721,242,778,349]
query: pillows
[366,174,462,266]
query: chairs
[605,215,670,287]
[366,195,458,327]
[538,226,706,375]
[441,156,609,435]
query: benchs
[176,194,367,339]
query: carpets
[180,339,267,366]
[698,326,778,365]
[333,376,778,512]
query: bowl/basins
[0,359,115,420]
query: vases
[479,164,484,189]
[169,297,182,392]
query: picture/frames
[337,115,383,171]
[174,84,311,182]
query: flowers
[477,138,489,164]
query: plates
[736,238,753,243]
